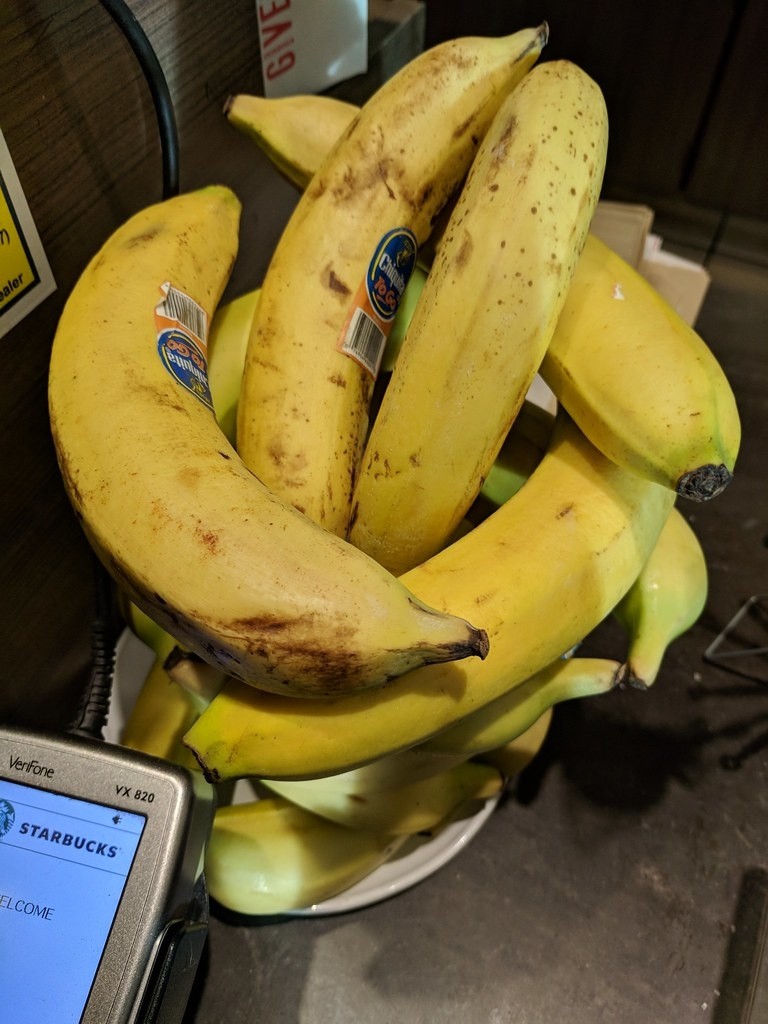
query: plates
[106,624,500,915]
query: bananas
[43,25,742,919]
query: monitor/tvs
[0,716,216,1023]
